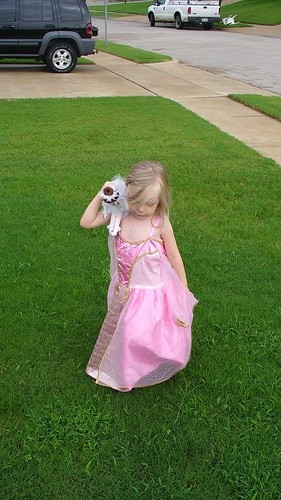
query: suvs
[0,0,99,73]
[148,0,221,30]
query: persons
[80,160,199,392]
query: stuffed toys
[101,173,130,237]
